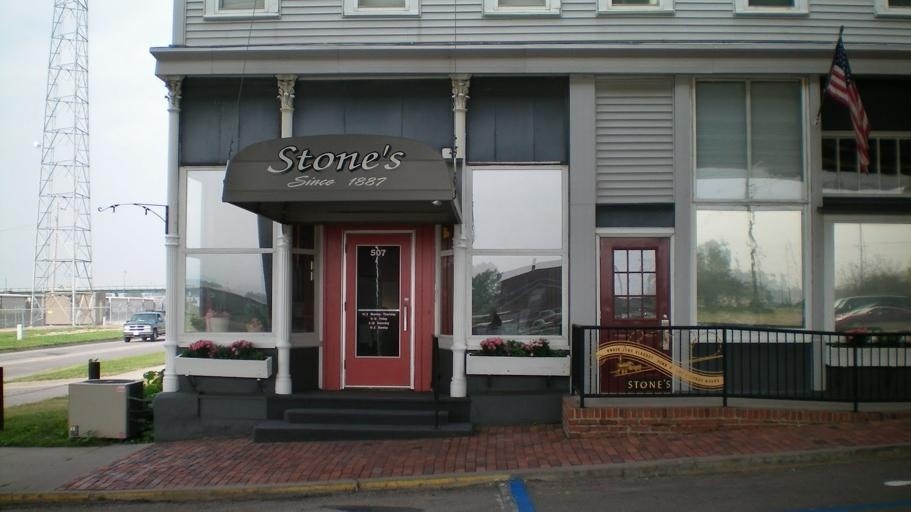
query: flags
[827,35,872,175]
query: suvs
[121,310,166,342]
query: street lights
[517,263,537,330]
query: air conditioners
[67,378,146,440]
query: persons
[486,307,503,333]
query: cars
[835,295,910,345]
[485,309,561,335]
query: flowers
[522,335,552,357]
[226,338,260,359]
[480,333,513,356]
[846,325,871,346]
[188,339,220,357]
